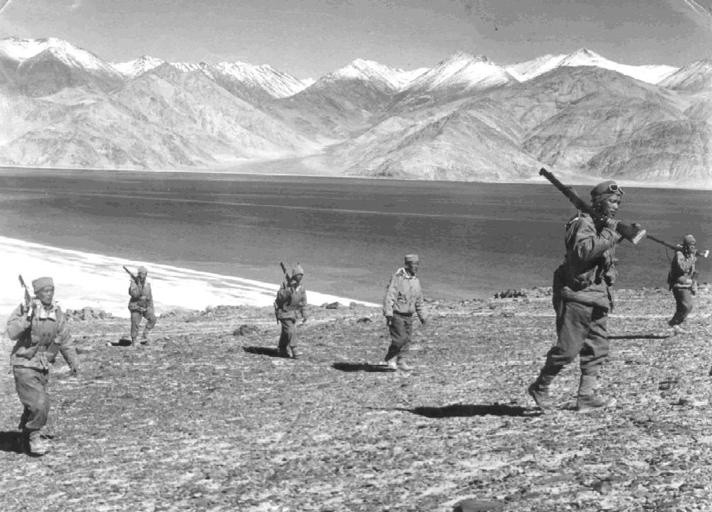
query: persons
[528,181,623,412]
[666,235,697,329]
[6,277,79,455]
[383,254,425,370]
[275,266,308,357]
[128,267,157,346]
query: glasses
[608,183,625,195]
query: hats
[138,265,148,273]
[404,253,419,263]
[683,233,696,246]
[291,263,304,277]
[589,180,623,207]
[31,275,55,294]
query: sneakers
[22,429,44,455]
[574,395,618,414]
[385,358,411,372]
[277,349,304,357]
[527,382,556,416]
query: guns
[538,166,647,246]
[123,265,144,298]
[18,275,36,346]
[645,232,710,259]
[280,262,294,306]
